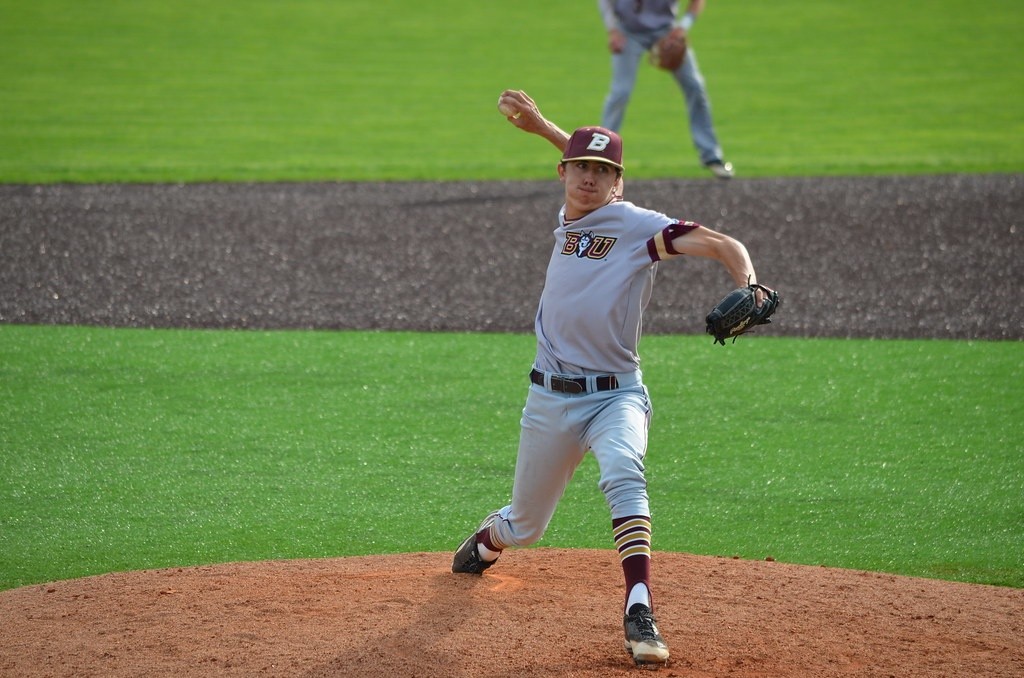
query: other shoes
[704,159,735,180]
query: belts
[531,368,617,394]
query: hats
[562,126,625,172]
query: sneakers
[623,603,669,664]
[452,510,502,575]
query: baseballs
[498,93,519,117]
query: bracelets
[679,16,693,31]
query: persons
[598,0,735,179]
[452,91,764,664]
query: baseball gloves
[650,28,688,71]
[703,272,781,347]
